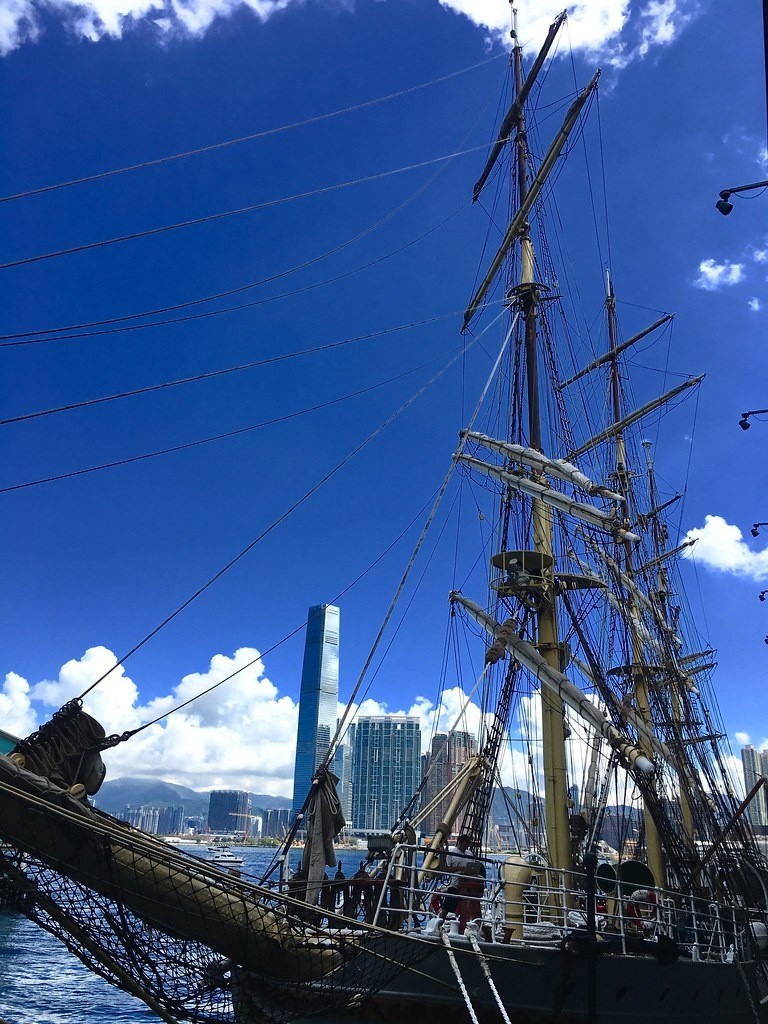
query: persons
[439,835,474,926]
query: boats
[208,844,230,852]
[207,852,244,866]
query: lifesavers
[626,889,659,932]
[430,887,462,918]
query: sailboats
[0,1,768,1024]
